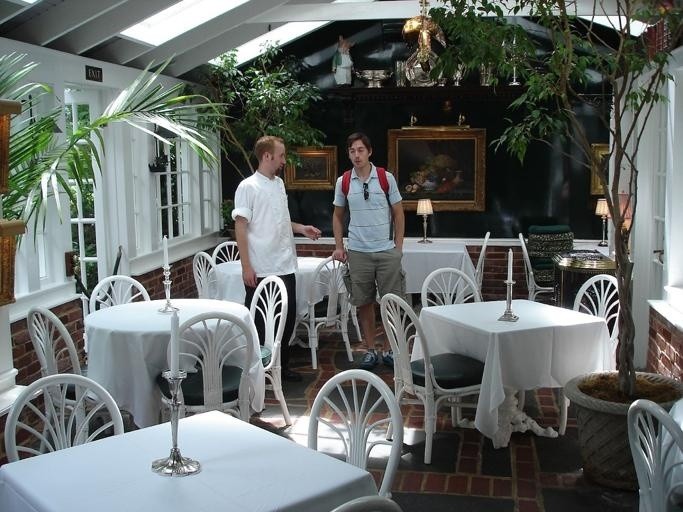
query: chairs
[154,311,251,427]
[299,256,355,370]
[5,373,125,461]
[473,232,491,293]
[308,368,405,497]
[90,274,148,309]
[379,292,485,465]
[517,231,553,301]
[350,308,364,343]
[573,272,620,342]
[26,307,137,453]
[422,267,483,425]
[193,251,222,299]
[626,398,683,510]
[210,241,240,265]
[249,275,295,427]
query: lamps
[414,197,434,245]
[592,195,613,248]
[613,187,635,259]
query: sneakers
[358,350,379,369]
[380,350,395,368]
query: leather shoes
[282,366,305,383]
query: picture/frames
[282,145,338,190]
[589,142,611,197]
[386,128,486,212]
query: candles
[167,310,186,379]
[161,234,170,268]
[507,247,512,282]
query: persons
[333,132,406,368]
[231,136,321,382]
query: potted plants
[418,0,683,492]
[0,44,240,312]
